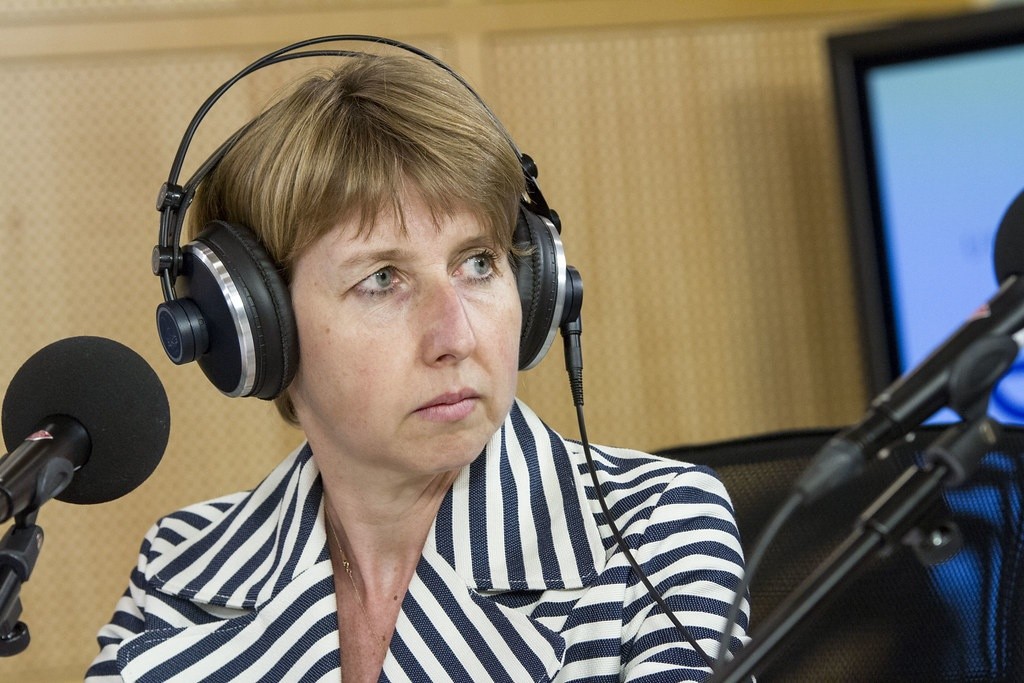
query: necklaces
[325,506,386,657]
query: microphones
[0,336,171,523]
[797,189,1024,506]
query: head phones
[151,34,586,403]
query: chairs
[658,419,1023,683]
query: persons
[81,53,755,683]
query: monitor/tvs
[827,0,1023,459]
